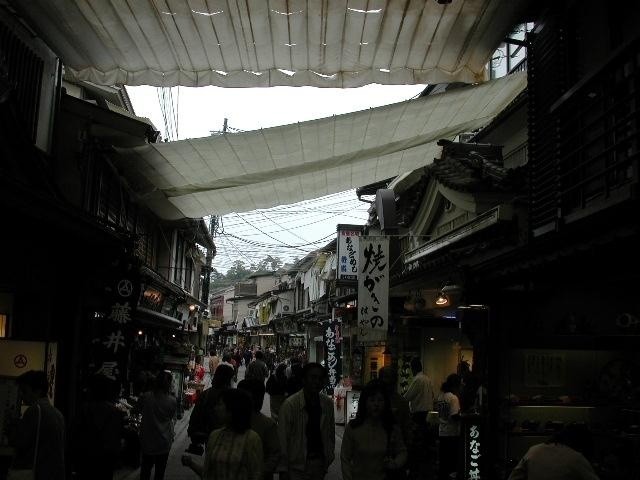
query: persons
[508,424,599,479]
[12,344,504,480]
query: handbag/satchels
[8,470,34,479]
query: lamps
[435,296,448,305]
